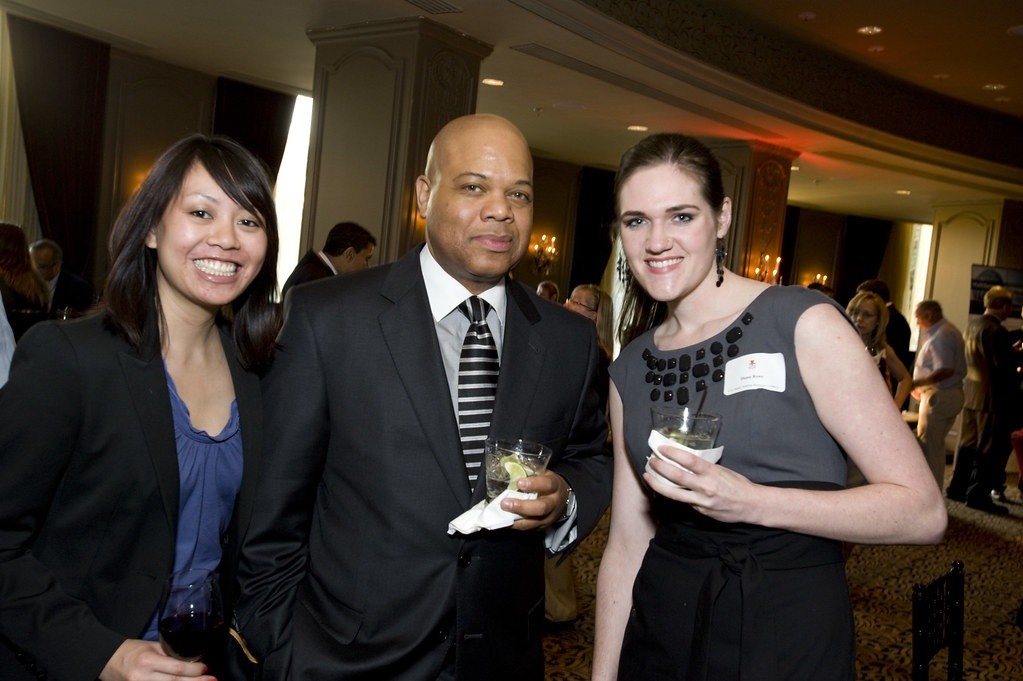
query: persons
[224,114,614,681]
[946,286,1023,516]
[591,133,947,681]
[910,299,967,491]
[808,282,834,298]
[282,221,377,295]
[536,281,559,304]
[0,224,85,387]
[0,134,281,681]
[855,280,911,413]
[563,284,613,359]
[846,291,913,410]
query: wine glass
[156,566,226,668]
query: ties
[456,296,500,495]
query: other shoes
[966,501,1009,516]
[945,491,966,502]
[991,488,1007,502]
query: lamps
[755,255,782,285]
[535,235,556,272]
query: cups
[650,405,722,466]
[865,345,877,360]
[485,437,553,510]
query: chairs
[1012,429,1023,483]
[911,560,965,681]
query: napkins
[645,431,725,489]
[446,491,538,535]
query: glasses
[566,298,597,314]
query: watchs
[554,487,576,522]
